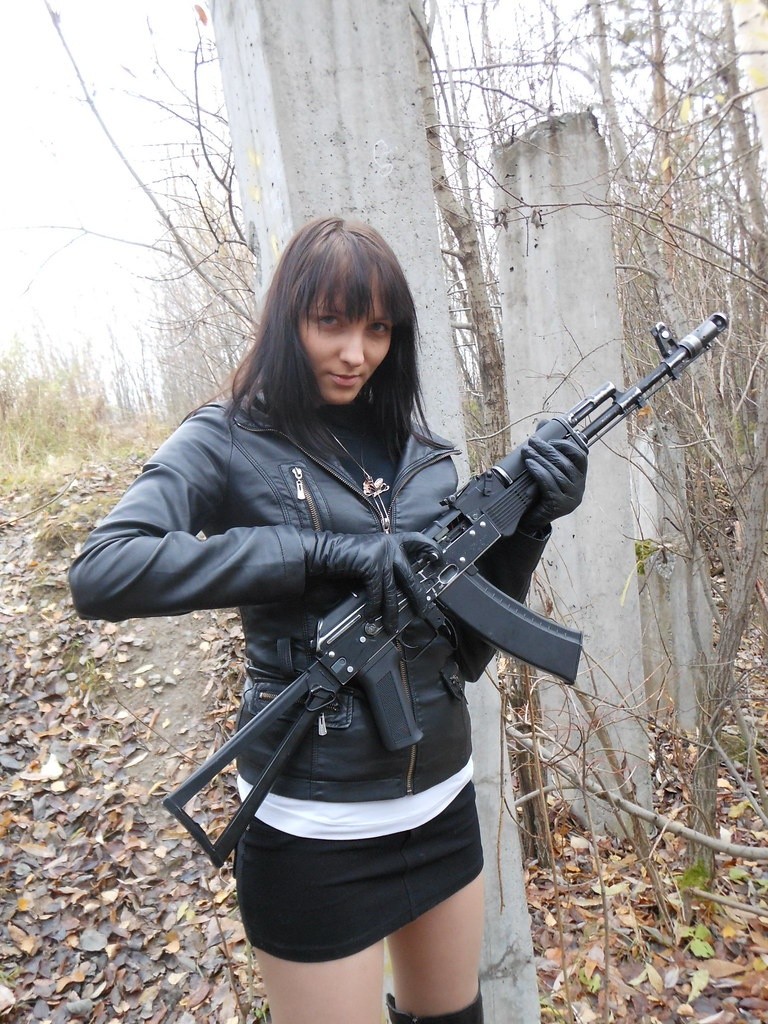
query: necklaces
[321,416,391,535]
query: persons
[67,215,589,1024]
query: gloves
[295,530,442,635]
[520,435,587,536]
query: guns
[162,309,732,870]
